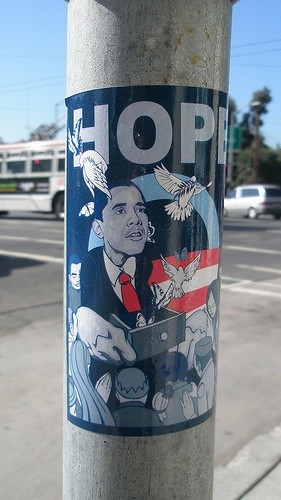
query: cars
[224,184,281,220]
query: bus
[0,138,66,222]
